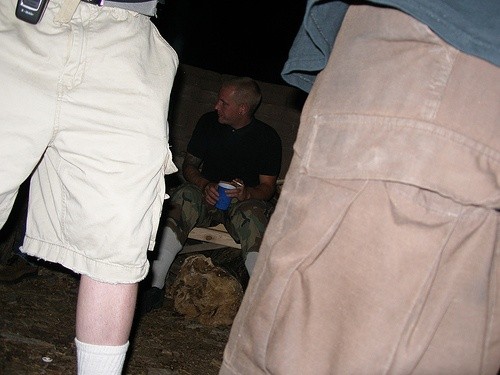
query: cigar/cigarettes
[232,180,243,187]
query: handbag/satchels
[169,254,244,327]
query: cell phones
[15,0,50,24]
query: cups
[214,183,237,211]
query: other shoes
[136,285,166,316]
[0,255,38,284]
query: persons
[0,0,179,375]
[220,1,499,374]
[135,77,282,307]
